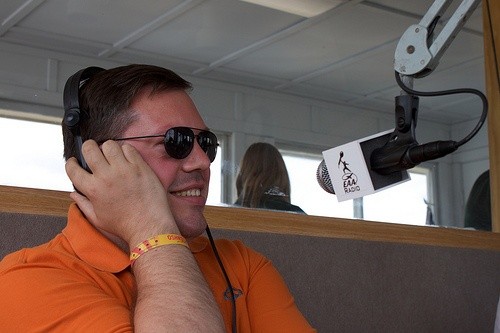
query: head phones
[62,66,107,199]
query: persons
[234,142,307,215]
[0,64,315,333]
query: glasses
[98,127,219,163]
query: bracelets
[129,233,190,270]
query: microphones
[317,129,459,202]
